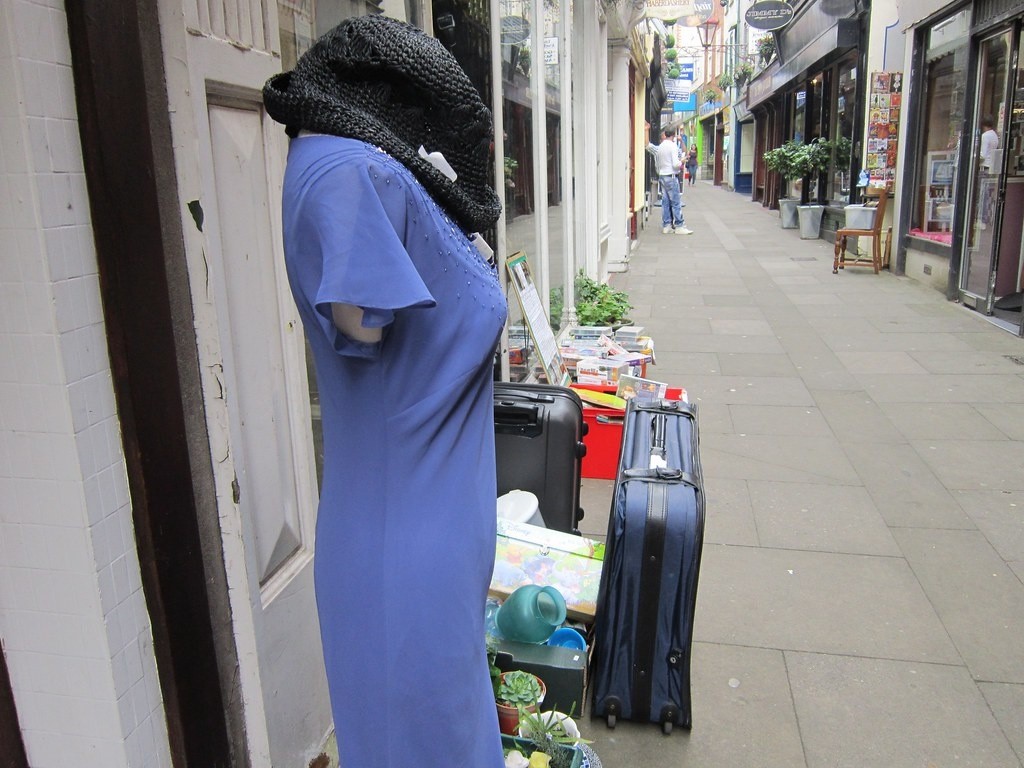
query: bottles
[483,583,588,657]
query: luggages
[590,396,706,736]
[493,381,589,536]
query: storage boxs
[487,628,596,720]
[506,324,688,482]
[843,205,876,230]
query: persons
[263,17,509,768]
[974,114,999,231]
[645,121,698,235]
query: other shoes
[692,184,696,187]
[688,182,690,187]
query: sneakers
[662,228,675,234]
[675,227,693,235]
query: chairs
[828,187,887,275]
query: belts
[667,174,678,177]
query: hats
[261,13,503,233]
[665,129,675,136]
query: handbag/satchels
[685,162,689,168]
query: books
[535,326,669,422]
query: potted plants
[763,144,804,229]
[785,140,832,240]
[493,670,545,734]
[513,696,593,752]
[501,734,582,768]
[574,267,633,330]
